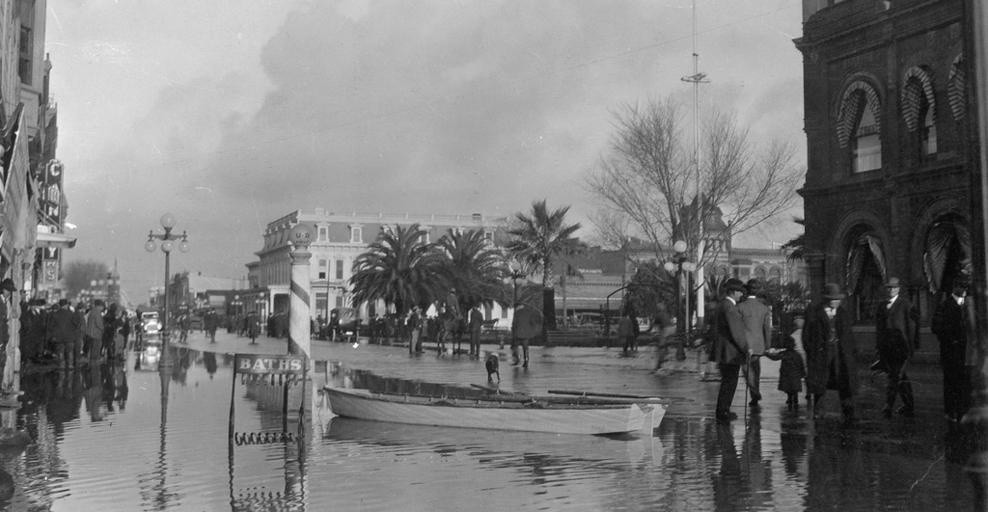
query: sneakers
[749,393,761,405]
[716,412,737,424]
[881,403,914,414]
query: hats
[449,287,456,294]
[782,337,796,348]
[722,278,765,293]
[820,283,845,300]
[883,277,903,288]
[25,298,69,309]
[0,278,17,292]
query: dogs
[485,355,501,384]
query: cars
[141,310,161,333]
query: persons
[738,278,773,404]
[203,309,209,337]
[874,276,916,416]
[177,311,188,343]
[48,363,130,421]
[764,336,806,404]
[467,304,483,355]
[712,279,747,420]
[227,309,322,346]
[366,305,428,355]
[510,300,534,367]
[22,297,130,363]
[801,282,861,421]
[446,288,460,314]
[1,276,18,382]
[208,306,218,343]
[933,271,975,422]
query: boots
[513,349,528,366]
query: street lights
[76,272,121,313]
[196,297,209,333]
[256,292,267,334]
[664,240,696,362]
[230,294,242,337]
[145,211,191,368]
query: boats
[322,417,665,466]
[319,381,668,436]
